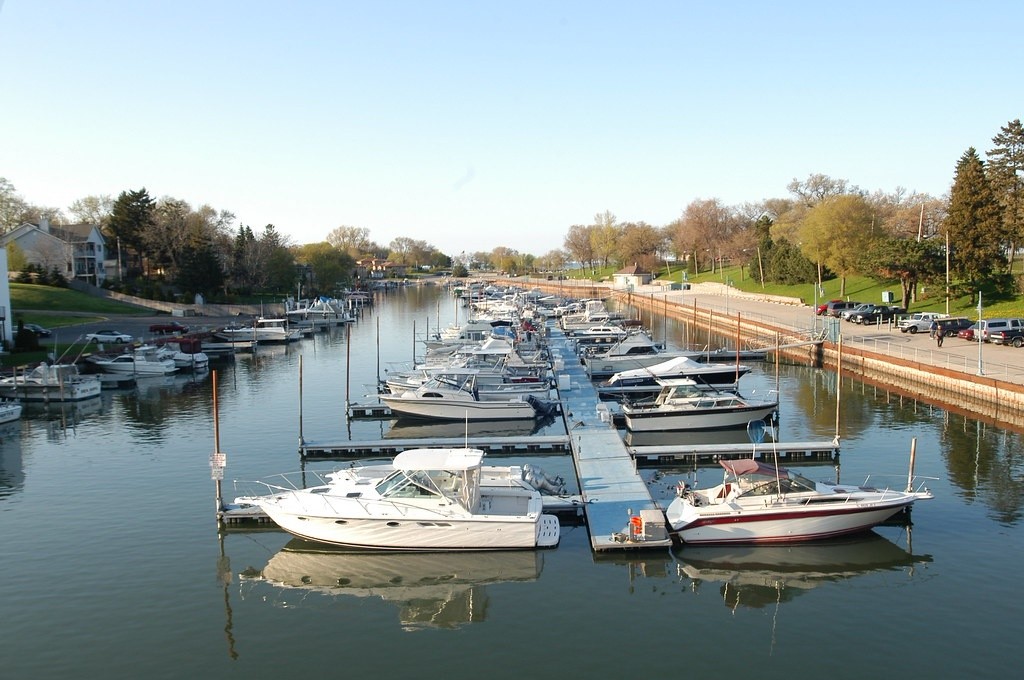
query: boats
[667,528,934,658]
[0,334,102,403]
[232,445,560,551]
[362,375,557,420]
[0,397,24,424]
[663,458,935,546]
[93,279,370,380]
[380,416,553,440]
[621,377,779,432]
[380,276,754,408]
[237,537,547,632]
[625,427,778,467]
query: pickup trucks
[991,328,1024,348]
[149,320,189,335]
[816,299,907,325]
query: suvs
[897,312,946,334]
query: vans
[934,318,975,337]
[973,318,1024,343]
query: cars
[85,329,134,344]
[11,323,53,341]
[958,324,975,341]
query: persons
[929,319,936,339]
[935,325,945,347]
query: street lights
[742,247,765,290]
[706,247,723,279]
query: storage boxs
[640,510,665,540]
[559,374,570,391]
[551,348,564,370]
[565,340,574,351]
[596,404,609,422]
